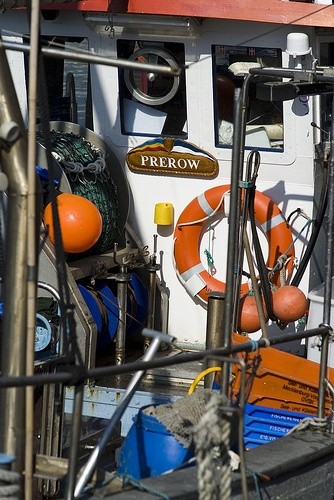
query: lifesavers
[173,185,295,304]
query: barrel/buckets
[117,405,194,480]
